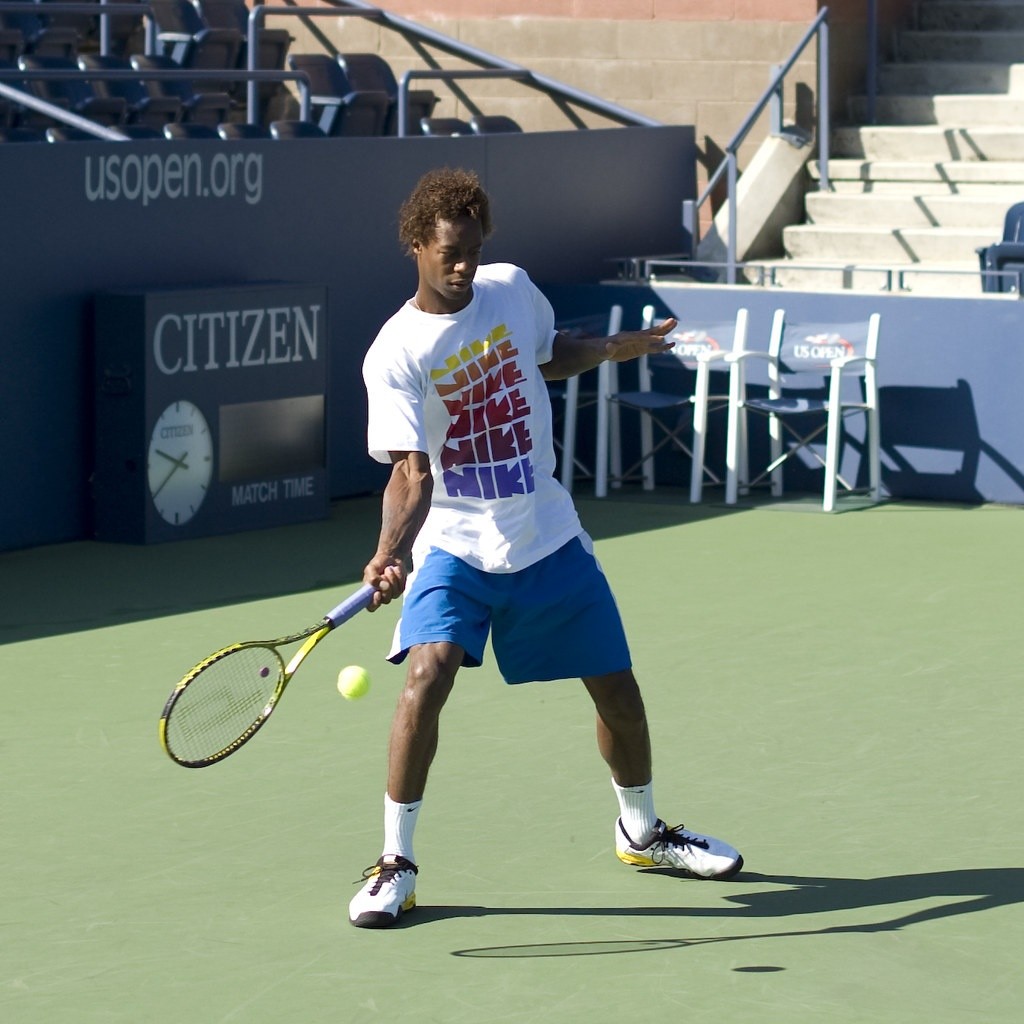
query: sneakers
[348,853,418,927]
[616,816,744,880]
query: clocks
[147,399,214,525]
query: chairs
[0,0,518,142]
[537,304,883,511]
[974,202,1024,293]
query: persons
[349,173,746,925]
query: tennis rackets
[157,583,378,769]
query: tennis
[337,665,371,701]
